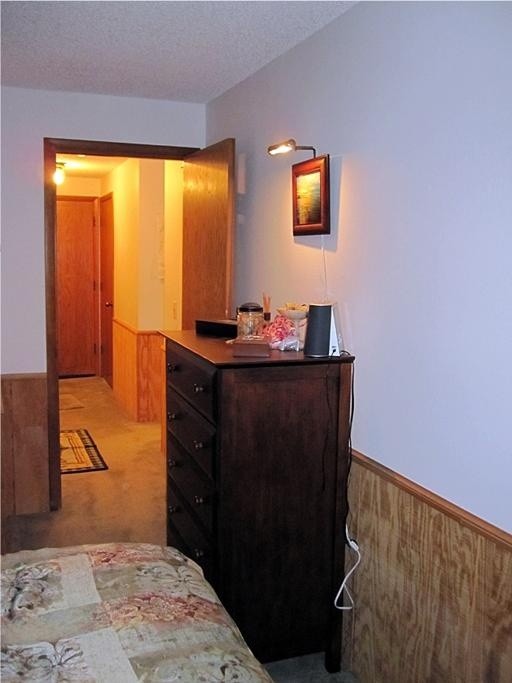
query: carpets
[59,392,86,408]
[59,428,109,474]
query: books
[233,333,270,357]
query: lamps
[267,137,318,158]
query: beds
[0,540,276,682]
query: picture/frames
[291,153,331,236]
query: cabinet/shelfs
[155,328,355,674]
